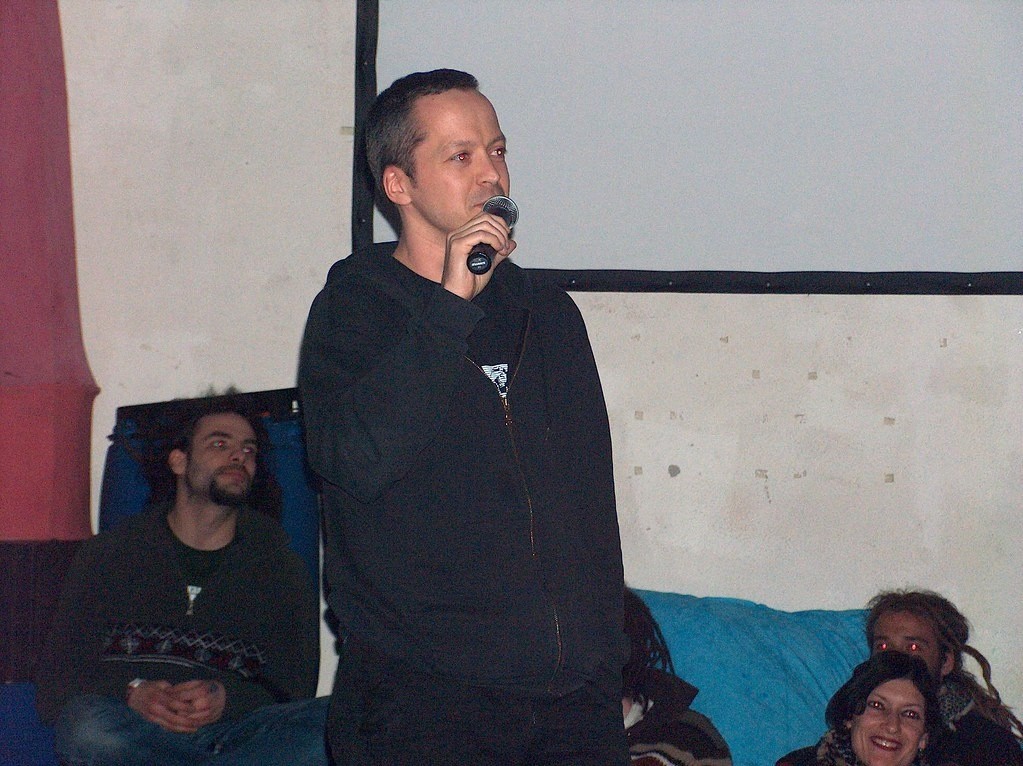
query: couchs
[624,587,875,766]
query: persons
[620,587,735,766]
[295,68,634,766]
[774,651,952,766]
[862,591,1023,766]
[36,396,332,766]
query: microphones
[466,194,519,275]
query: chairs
[0,388,319,766]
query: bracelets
[123,678,146,699]
